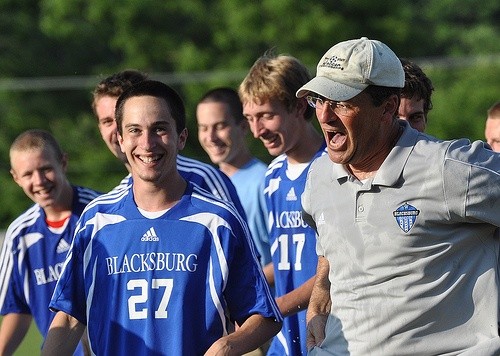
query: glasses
[304,95,375,116]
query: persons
[396,58,434,133]
[296,37,500,356]
[0,47,328,356]
[484,102,500,153]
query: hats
[296,37,406,101]
